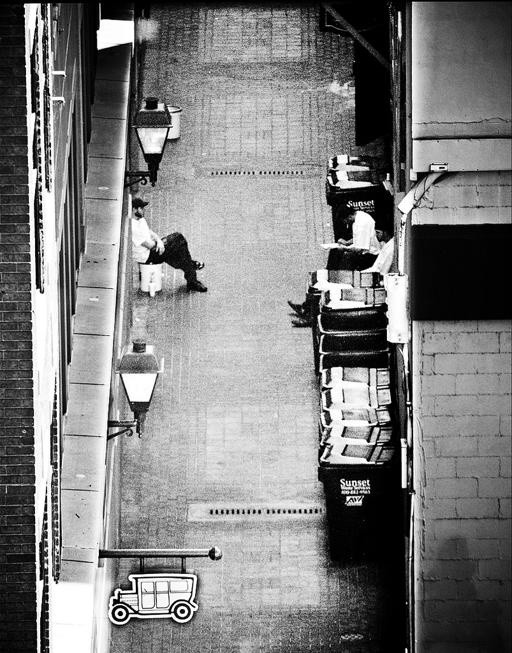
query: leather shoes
[287,299,306,314]
[192,260,204,269]
[186,281,208,293]
[292,317,315,327]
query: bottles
[147,272,156,297]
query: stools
[135,259,167,299]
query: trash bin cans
[325,154,386,247]
[166,105,183,141]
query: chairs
[305,234,399,327]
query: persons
[322,204,380,271]
[286,218,397,325]
[132,197,208,292]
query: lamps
[108,334,164,441]
[123,89,174,193]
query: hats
[132,197,149,207]
